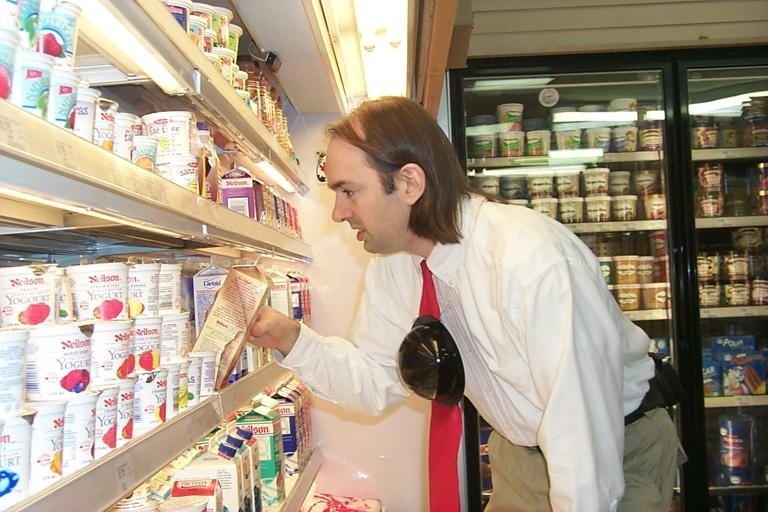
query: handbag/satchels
[624,360,681,426]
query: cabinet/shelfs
[0,0,367,512]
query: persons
[204,95,683,512]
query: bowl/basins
[470,96,767,311]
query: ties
[419,260,463,512]
